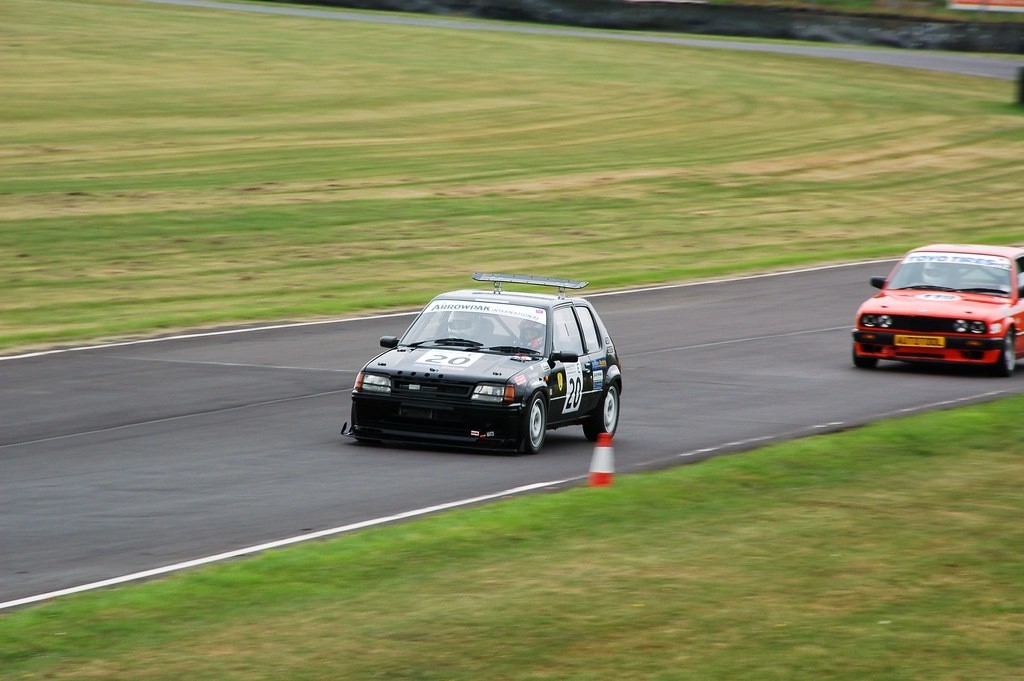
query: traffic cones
[586,433,616,489]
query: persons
[976,266,1012,290]
[910,262,955,288]
[511,319,554,353]
[421,309,486,349]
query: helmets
[518,320,544,349]
[448,312,481,337]
[923,263,949,283]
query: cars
[851,241,1024,377]
[349,271,621,453]
